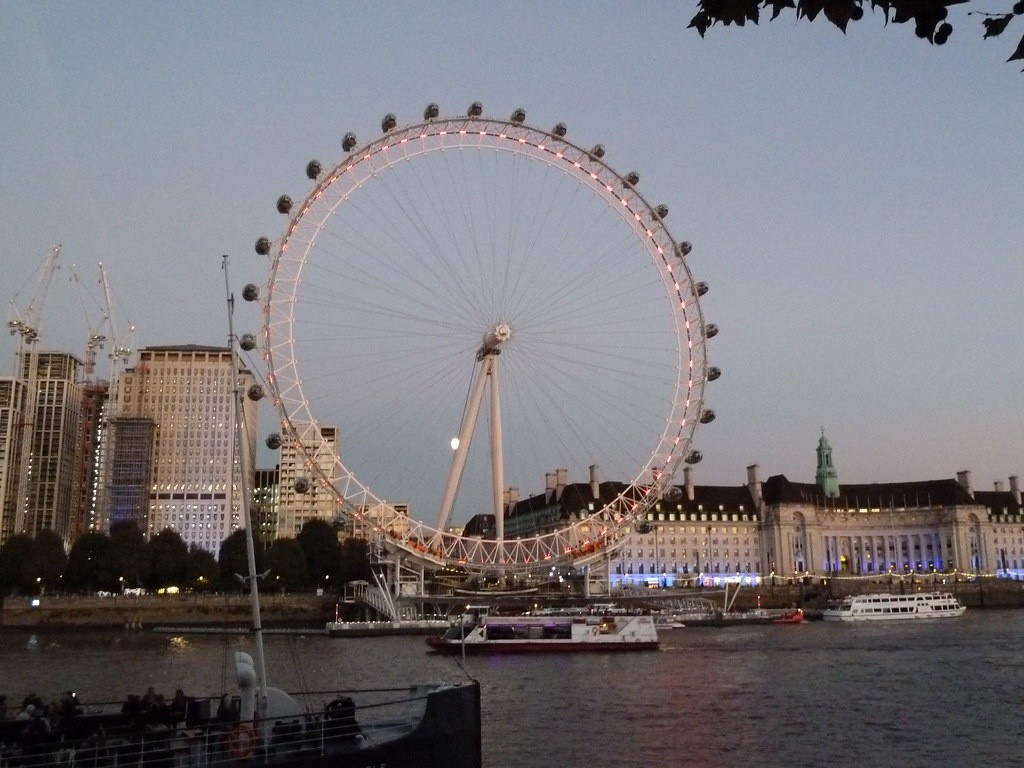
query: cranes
[6,237,136,549]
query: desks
[0,706,175,749]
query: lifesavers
[225,726,255,758]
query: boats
[673,609,804,626]
[819,591,968,621]
[425,602,661,653]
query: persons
[1,687,323,768]
